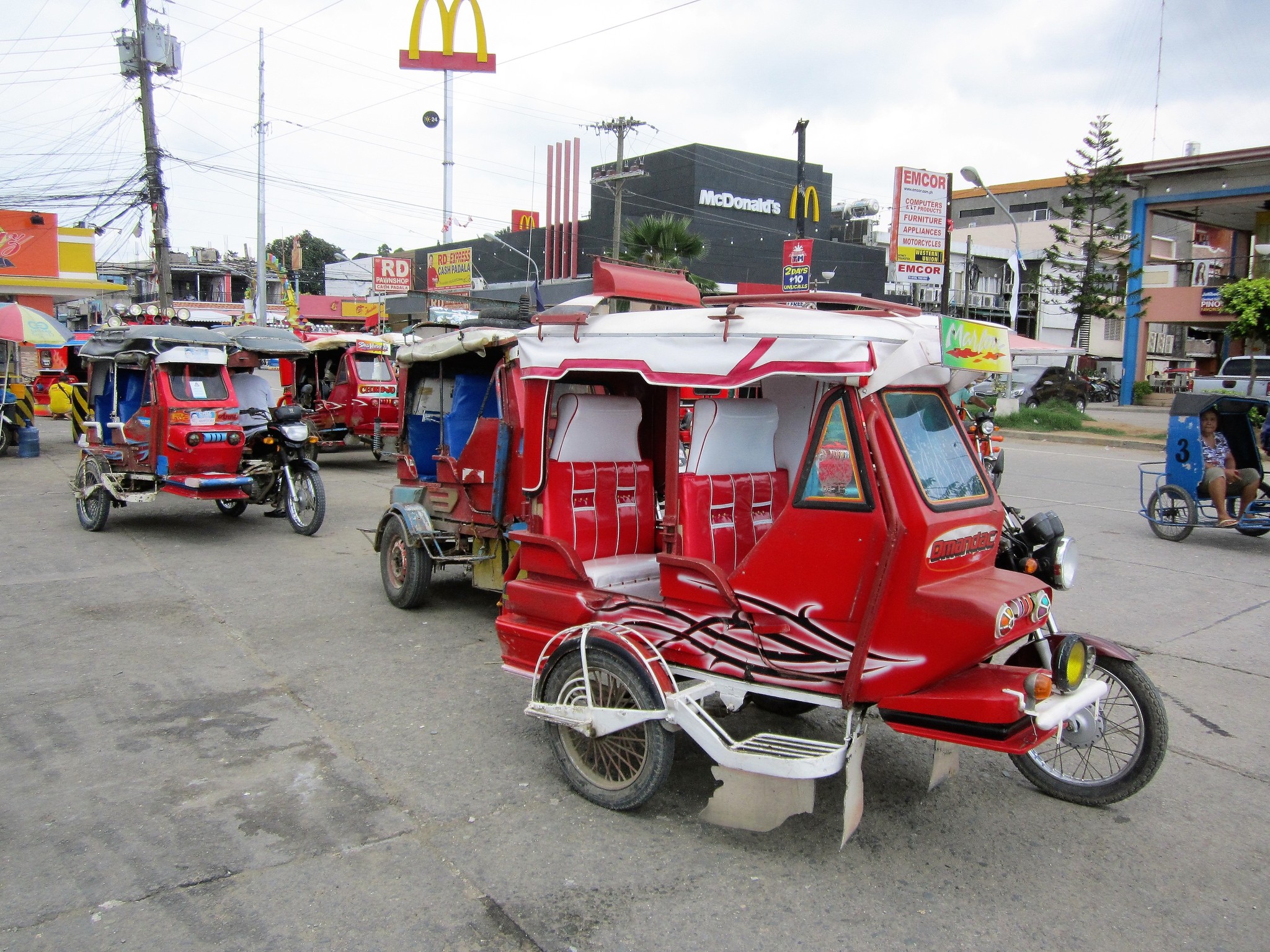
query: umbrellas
[0,302,76,403]
[237,312,287,324]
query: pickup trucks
[1192,355,1270,396]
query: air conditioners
[887,289,897,295]
[899,291,908,295]
[932,290,940,302]
[972,284,1037,309]
[1112,274,1119,282]
[918,288,924,301]
[472,278,484,290]
[1033,209,1051,221]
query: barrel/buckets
[11,384,25,399]
[243,299,255,313]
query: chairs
[94,370,126,445]
[680,398,788,578]
[1149,373,1186,393]
[546,396,665,598]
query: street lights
[484,232,539,306]
[960,165,1020,331]
[334,253,381,335]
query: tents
[175,310,232,327]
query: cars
[968,364,1090,414]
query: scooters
[1079,370,1122,403]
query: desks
[1155,379,1175,392]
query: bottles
[18,420,40,457]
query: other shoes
[264,508,287,517]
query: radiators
[120,375,150,444]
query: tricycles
[1137,392,1270,542]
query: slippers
[51,417,59,420]
[1214,519,1239,527]
[64,417,72,420]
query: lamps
[73,221,122,236]
[1255,244,1270,260]
[30,210,45,225]
[352,293,368,298]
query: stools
[1198,483,1239,497]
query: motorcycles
[490,254,1169,812]
[277,333,423,463]
[67,325,326,535]
[356,294,542,609]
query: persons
[384,324,392,332]
[372,327,379,336]
[220,366,278,450]
[300,351,377,399]
[1199,407,1267,526]
[264,470,303,517]
[428,254,439,288]
[950,387,996,416]
[48,375,73,420]
